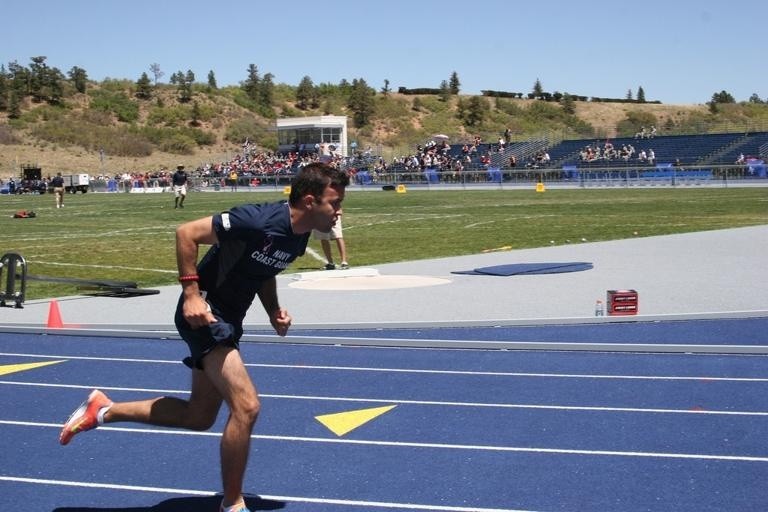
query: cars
[0,180,22,194]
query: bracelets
[177,275,199,282]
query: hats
[177,164,185,168]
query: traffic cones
[45,299,64,329]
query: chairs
[385,131,768,181]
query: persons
[59,161,350,512]
[379,129,551,183]
[734,152,745,164]
[579,126,657,165]
[313,214,348,270]
[53,172,65,209]
[7,175,53,194]
[88,150,366,191]
[171,163,188,209]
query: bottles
[595,299,604,316]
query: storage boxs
[607,290,638,315]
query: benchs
[280,155,378,175]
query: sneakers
[60,388,113,445]
[321,264,336,270]
[340,264,348,270]
